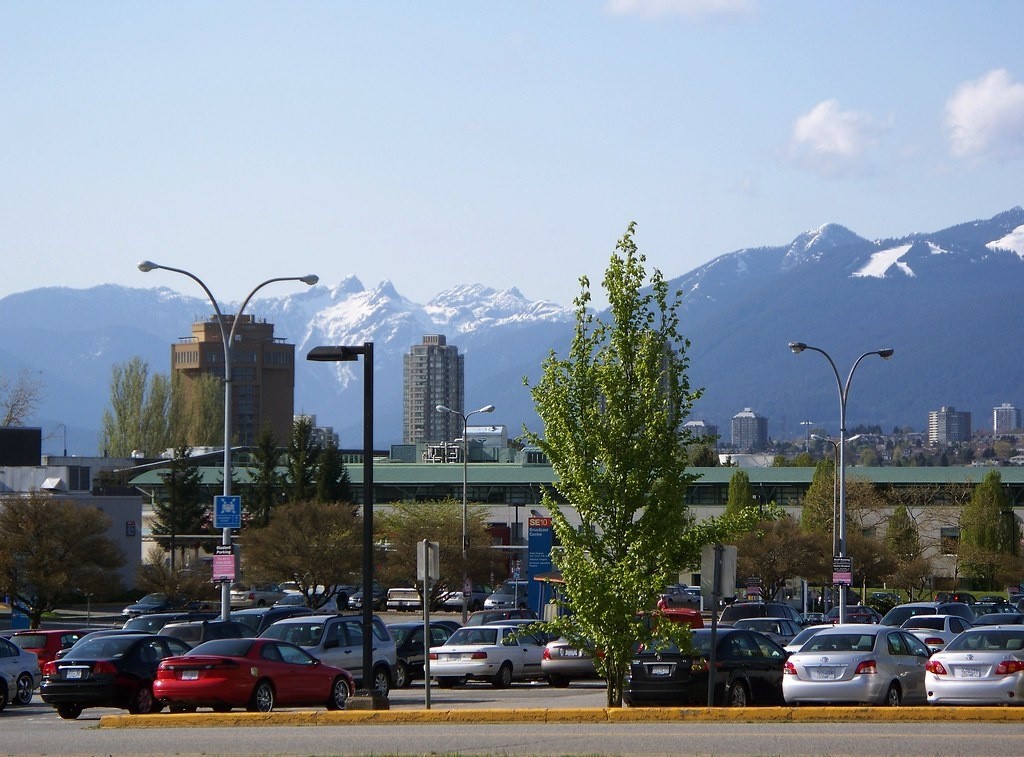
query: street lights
[304,342,377,699]
[157,466,175,577]
[810,434,863,609]
[137,258,319,620]
[999,510,1015,556]
[751,493,764,543]
[507,501,519,576]
[787,341,896,625]
[436,402,497,624]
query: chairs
[1006,639,1023,650]
[835,638,853,651]
[964,640,982,650]
[433,631,444,642]
[143,645,158,660]
[291,629,303,642]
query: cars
[0,581,1024,698]
[619,627,793,708]
[0,667,19,714]
[0,635,42,707]
[781,624,942,707]
[39,633,193,720]
[925,624,1023,707]
[151,637,356,714]
